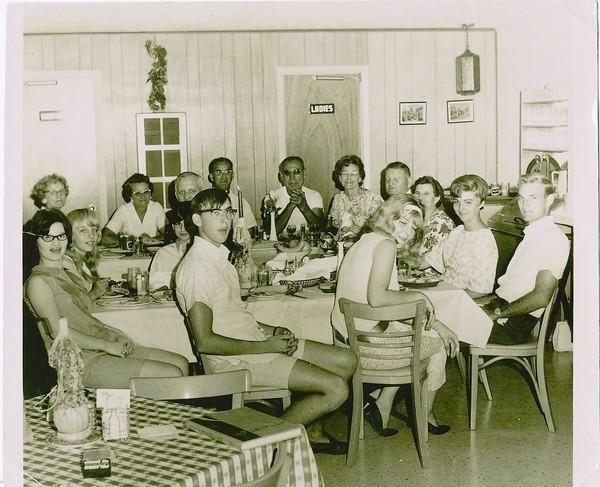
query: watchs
[494,304,501,317]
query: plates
[96,241,444,307]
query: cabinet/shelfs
[518,88,569,200]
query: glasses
[211,169,233,176]
[42,189,69,199]
[197,208,237,220]
[131,188,151,198]
[284,168,303,176]
[23,232,69,242]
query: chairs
[35,318,295,419]
[466,281,557,435]
[339,299,426,469]
[127,368,250,411]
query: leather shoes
[308,432,349,454]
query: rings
[433,310,435,314]
[124,351,127,356]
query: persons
[326,154,385,248]
[330,192,461,437]
[479,174,570,345]
[208,157,258,239]
[24,173,70,232]
[383,161,412,195]
[149,207,199,290]
[410,176,456,276]
[99,173,170,246]
[174,172,204,201]
[174,188,359,454]
[62,204,110,301]
[263,156,325,239]
[417,174,500,300]
[24,212,189,389]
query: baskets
[280,271,335,289]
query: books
[186,407,303,451]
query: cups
[118,223,348,302]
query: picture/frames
[447,99,473,124]
[399,101,427,125]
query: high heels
[364,401,398,437]
[428,422,451,436]
